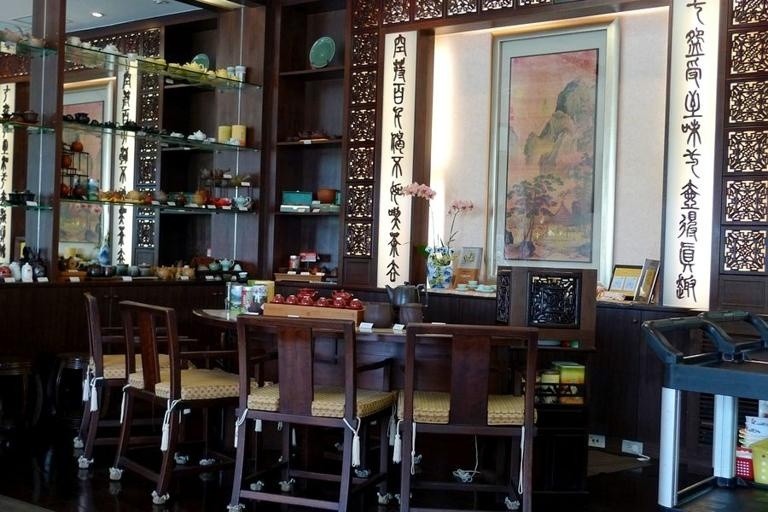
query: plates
[309,36,336,69]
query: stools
[0,355,111,434]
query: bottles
[21,262,33,283]
[88,264,151,277]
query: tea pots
[332,289,353,304]
[61,35,254,85]
[385,281,429,312]
[59,248,242,271]
[1,30,47,48]
[60,112,248,153]
[297,128,329,139]
[62,139,254,213]
[295,285,319,302]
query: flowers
[404,182,475,286]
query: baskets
[736,447,753,481]
[750,438,768,484]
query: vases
[424,249,455,289]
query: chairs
[116,298,259,508]
[229,309,399,512]
[388,322,541,510]
[72,292,200,475]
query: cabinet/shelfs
[1,0,272,419]
[266,1,352,290]
[588,305,702,466]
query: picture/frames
[608,257,660,305]
[483,15,623,285]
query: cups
[273,294,362,311]
[365,301,427,330]
[239,272,248,279]
[458,280,497,291]
[288,137,297,142]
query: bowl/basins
[318,188,336,203]
[19,194,35,206]
[9,193,19,205]
[20,113,38,122]
